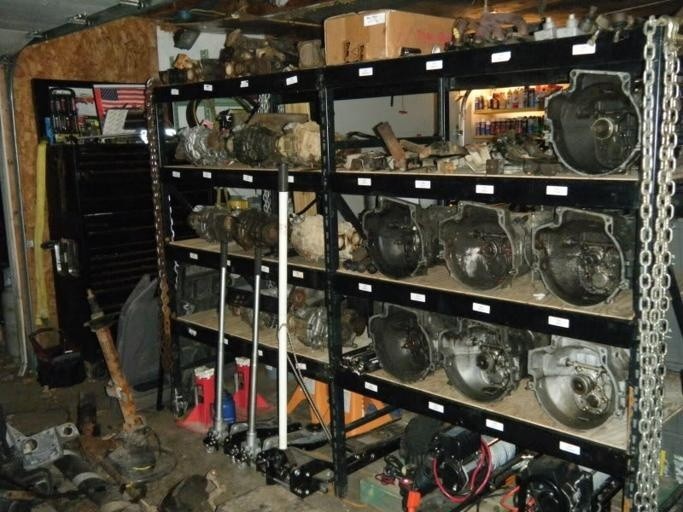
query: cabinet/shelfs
[145,21,683,512]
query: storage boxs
[322,9,457,66]
